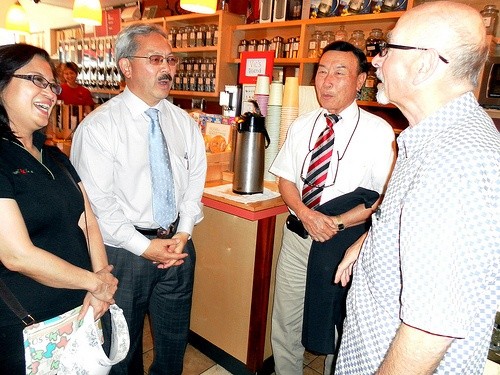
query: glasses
[12,75,62,95]
[123,54,179,67]
[299,149,339,187]
[374,38,448,65]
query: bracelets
[336,214,344,231]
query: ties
[302,114,342,211]
[147,108,178,230]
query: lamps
[73,0,102,27]
[180,0,218,15]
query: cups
[250,75,299,184]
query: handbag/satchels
[22,304,105,375]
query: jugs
[232,112,266,194]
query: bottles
[480,5,500,36]
[306,25,386,101]
[167,24,219,48]
[238,36,300,59]
[170,56,217,92]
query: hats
[56,302,130,375]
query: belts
[136,216,180,235]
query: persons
[334,0,500,375]
[70,22,207,375]
[56,62,92,111]
[0,43,119,375]
[268,41,397,375]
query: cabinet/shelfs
[48,0,422,102]
